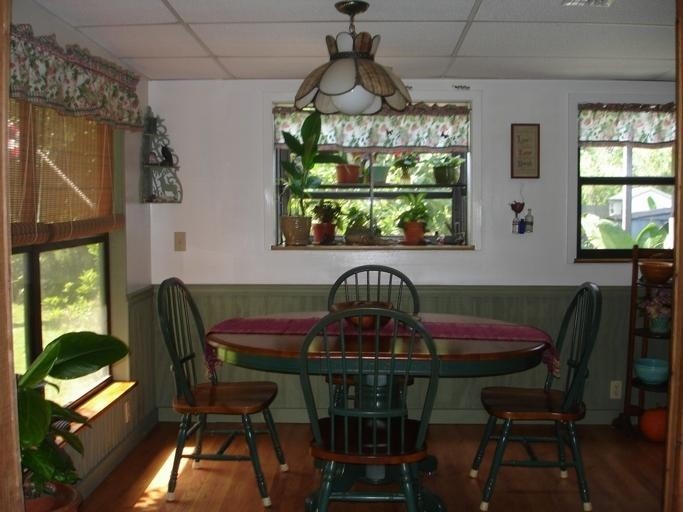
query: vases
[648,313,669,337]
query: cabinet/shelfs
[139,105,183,204]
[620,245,674,439]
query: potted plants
[16,330,129,512]
[272,112,465,246]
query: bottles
[512,208,534,234]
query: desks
[207,311,551,512]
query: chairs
[304,265,448,512]
[158,277,290,508]
[469,282,602,512]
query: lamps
[294,0,412,116]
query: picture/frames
[510,123,540,179]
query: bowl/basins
[639,261,674,284]
[635,359,670,388]
[328,301,396,330]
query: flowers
[636,287,673,320]
